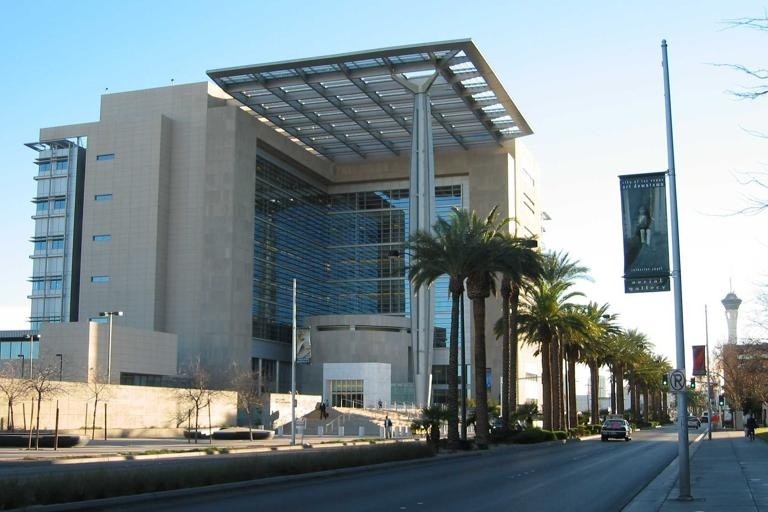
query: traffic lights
[692,378,695,389]
[662,374,668,386]
[719,396,724,406]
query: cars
[687,409,709,431]
[599,418,633,442]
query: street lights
[18,354,24,379]
[98,311,125,384]
[55,354,64,381]
[24,334,42,377]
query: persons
[378,401,382,408]
[384,416,392,439]
[746,413,757,441]
[631,205,655,247]
[320,403,326,420]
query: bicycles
[746,429,754,442]
